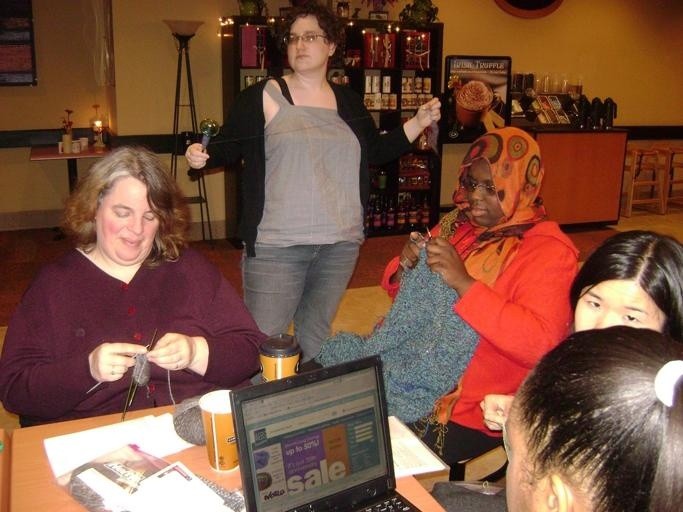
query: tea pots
[579,95,617,129]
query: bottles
[366,195,431,229]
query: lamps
[161,18,214,245]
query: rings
[402,258,408,265]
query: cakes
[458,77,494,126]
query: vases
[61,133,70,153]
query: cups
[533,73,570,93]
[198,389,240,472]
[80,138,89,150]
[259,333,302,385]
[376,169,387,189]
[73,140,80,153]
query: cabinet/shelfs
[220,15,446,247]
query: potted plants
[237,0,269,17]
[399,0,440,25]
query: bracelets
[184,335,196,371]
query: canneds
[337,1,349,17]
[402,75,434,109]
[364,75,398,110]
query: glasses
[463,179,495,194]
[284,31,329,42]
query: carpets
[287,283,393,337]
[606,208,682,247]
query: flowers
[61,108,72,134]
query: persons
[504,326,682,512]
[183,3,447,365]
[0,144,272,431]
[365,128,583,486]
[477,226,683,436]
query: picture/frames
[496,0,564,20]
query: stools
[619,148,666,218]
[652,143,683,212]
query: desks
[28,142,109,240]
[11,399,453,512]
[612,125,683,205]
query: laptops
[229,354,422,512]
